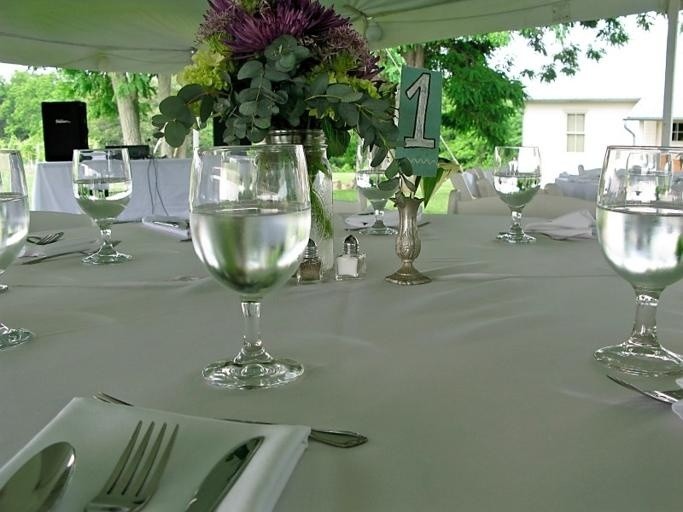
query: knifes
[181,431,266,512]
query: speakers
[42,101,89,162]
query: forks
[26,230,64,245]
[82,418,181,512]
[606,376,683,407]
[19,238,122,266]
[91,389,367,451]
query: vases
[256,128,335,287]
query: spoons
[0,441,78,512]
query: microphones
[147,138,153,159]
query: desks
[0,213,683,512]
[33,159,221,221]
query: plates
[342,211,424,229]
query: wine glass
[354,142,400,239]
[491,144,543,245]
[186,141,313,392]
[590,141,683,376]
[0,148,34,353]
[71,148,135,266]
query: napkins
[672,377,682,421]
[11,233,99,258]
[345,205,421,229]
[0,396,312,510]
[524,209,598,241]
[141,214,208,236]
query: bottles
[297,232,369,284]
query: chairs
[1,150,29,226]
[521,165,683,214]
[447,167,510,216]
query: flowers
[148,0,417,279]
